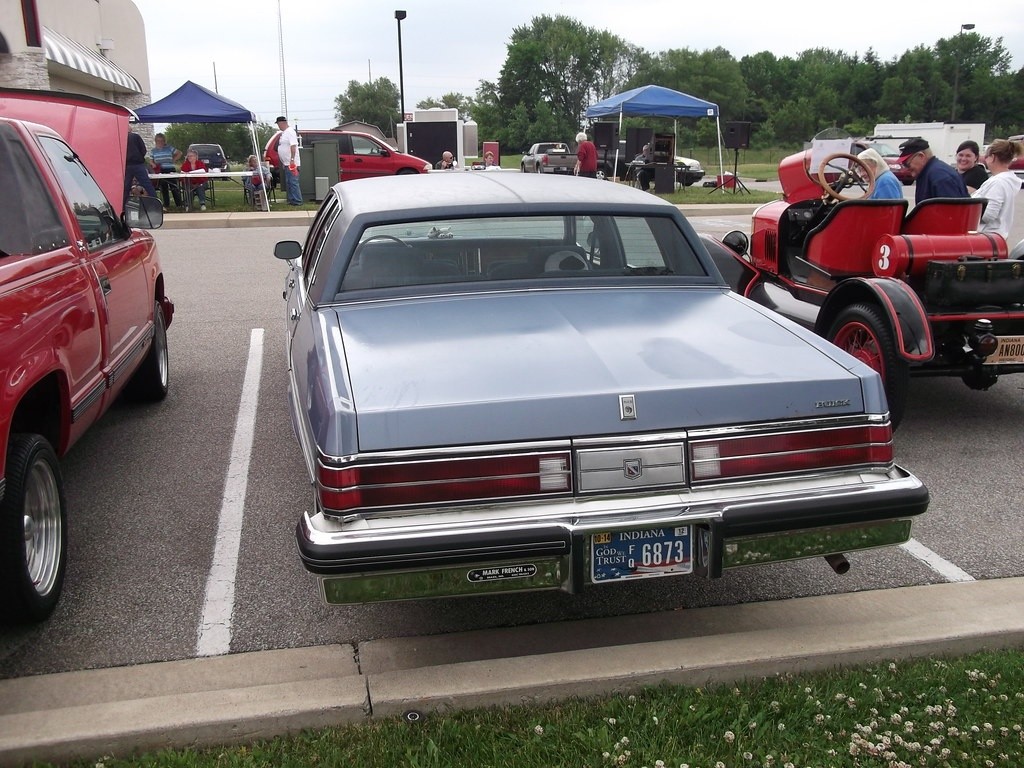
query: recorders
[471,162,486,170]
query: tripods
[708,149,751,196]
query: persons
[480,151,497,170]
[895,138,971,205]
[857,148,903,199]
[122,124,167,211]
[635,144,655,192]
[182,146,209,212]
[274,117,303,206]
[956,140,989,197]
[435,151,453,170]
[242,155,272,206]
[971,139,1022,258]
[149,134,185,209]
[575,132,599,179]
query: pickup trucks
[520,143,577,174]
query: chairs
[359,248,421,290]
[242,160,276,204]
[179,177,216,208]
[801,200,908,288]
[903,197,989,236]
[529,246,591,275]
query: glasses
[899,151,925,168]
[449,155,454,159]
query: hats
[275,117,286,123]
[894,136,929,164]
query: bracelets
[291,157,294,161]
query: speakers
[725,121,751,149]
[625,128,654,163]
[654,133,675,193]
[593,121,619,149]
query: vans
[263,130,432,189]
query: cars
[697,127,1024,431]
[273,169,932,606]
[0,87,174,622]
[858,139,915,186]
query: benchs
[350,237,577,277]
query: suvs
[595,141,705,186]
[184,143,230,181]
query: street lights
[952,23,975,123]
[394,11,408,123]
[294,119,299,137]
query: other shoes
[200,204,206,211]
[163,206,169,211]
[186,206,188,212]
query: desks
[147,172,253,212]
[624,162,690,192]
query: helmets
[545,249,589,273]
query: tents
[129,81,270,212]
[585,85,724,195]
